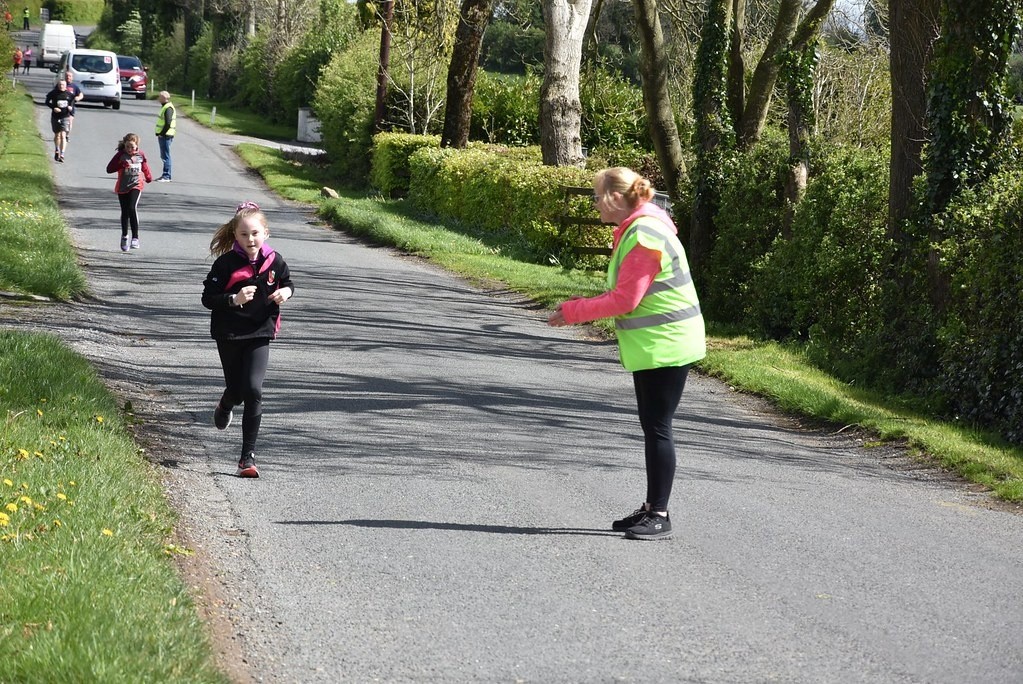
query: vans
[33,20,76,69]
[50,49,122,110]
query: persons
[24,6,31,29]
[22,46,32,76]
[14,45,22,76]
[3,10,11,30]
[106,133,153,252]
[156,91,176,181]
[547,166,706,539]
[45,72,83,161]
[200,201,294,477]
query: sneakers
[234,459,261,478]
[66,136,71,144]
[612,503,650,532]
[130,237,141,249]
[55,152,60,162]
[624,510,674,541]
[155,175,173,183]
[120,234,130,253]
[213,400,234,433]
[58,156,65,162]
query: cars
[116,54,150,100]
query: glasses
[593,194,611,203]
